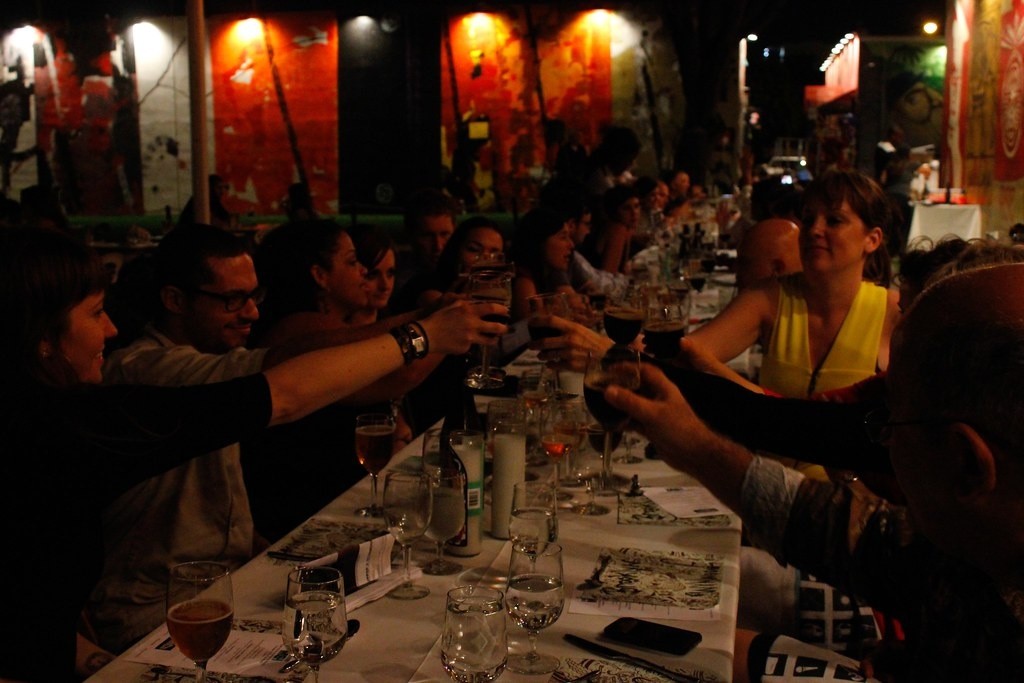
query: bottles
[443,426,482,557]
[437,367,483,469]
[492,419,527,541]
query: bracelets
[411,321,429,353]
[390,320,426,366]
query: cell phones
[603,617,703,657]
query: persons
[0,67,1024,683]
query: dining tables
[84,250,749,683]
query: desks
[908,202,984,250]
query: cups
[440,585,509,683]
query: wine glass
[507,483,559,582]
[419,471,467,576]
[380,472,430,599]
[282,566,350,683]
[167,560,235,683]
[503,538,565,674]
[464,189,750,515]
[354,411,396,518]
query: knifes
[564,633,700,683]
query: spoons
[279,618,362,674]
